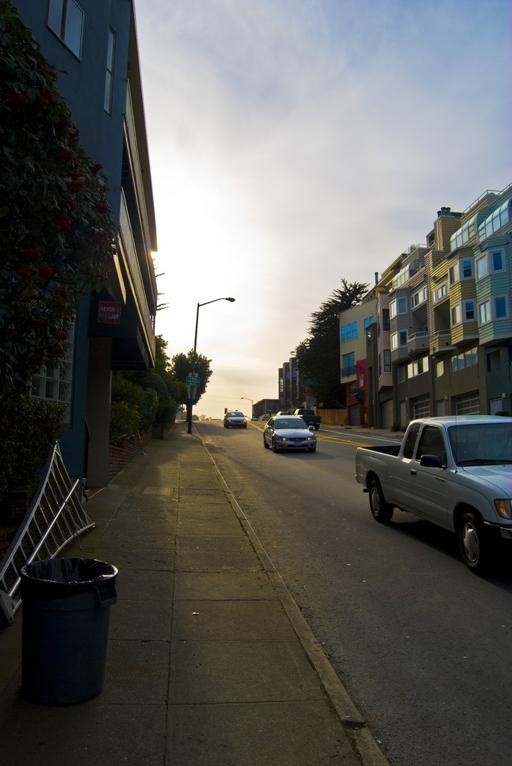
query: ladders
[0,438,95,626]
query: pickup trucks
[355,414,512,569]
[294,408,321,430]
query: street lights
[241,397,253,417]
[187,296,236,435]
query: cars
[224,410,247,427]
[276,411,291,414]
[263,414,317,452]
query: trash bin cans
[21,557,120,707]
